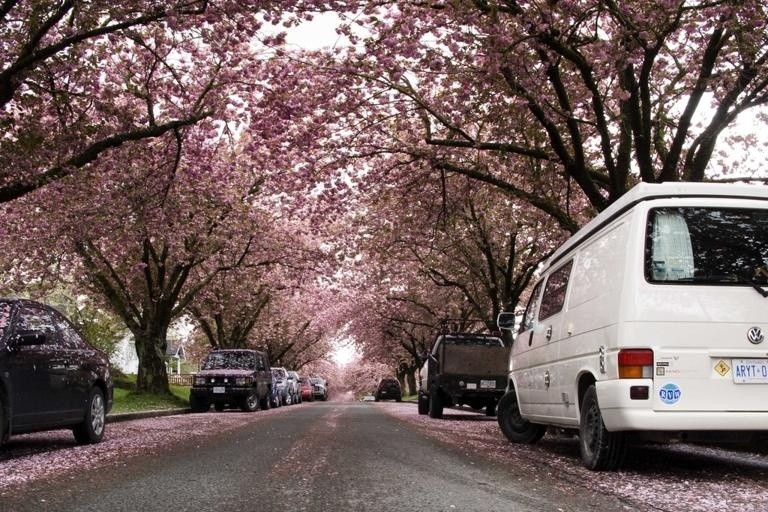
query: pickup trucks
[416,333,509,418]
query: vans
[497,182,768,472]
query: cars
[0,298,114,445]
[360,396,375,402]
[270,367,329,408]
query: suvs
[189,349,272,412]
[374,377,402,402]
